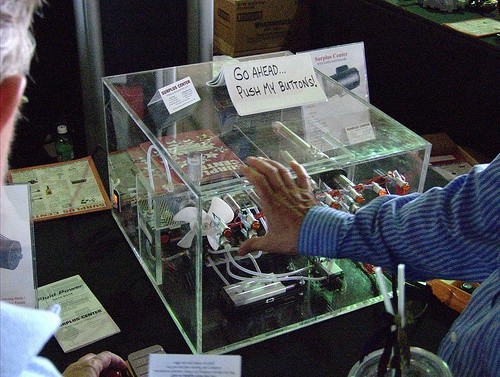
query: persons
[0,0,131,377]
[237,154,500,377]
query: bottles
[56,125,75,163]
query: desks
[32,147,459,377]
[313,0,500,158]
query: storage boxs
[213,0,311,58]
[100,49,433,356]
